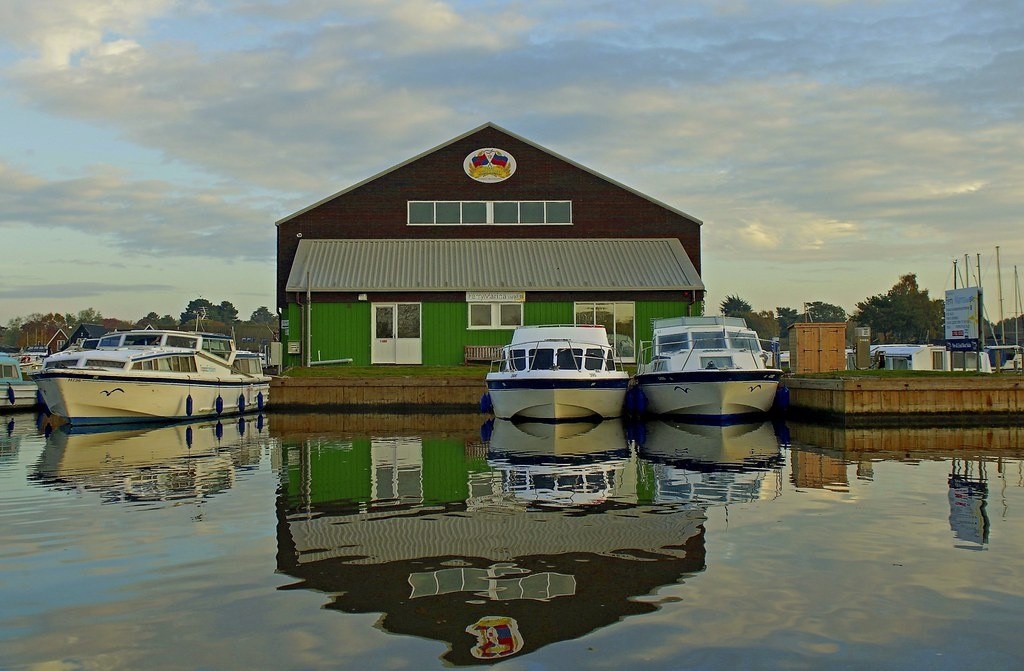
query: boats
[0,356,40,409]
[635,315,783,425]
[638,420,787,473]
[485,324,633,424]
[487,417,632,512]
[34,410,269,501]
[29,330,272,425]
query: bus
[984,345,1023,370]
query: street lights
[953,261,957,289]
